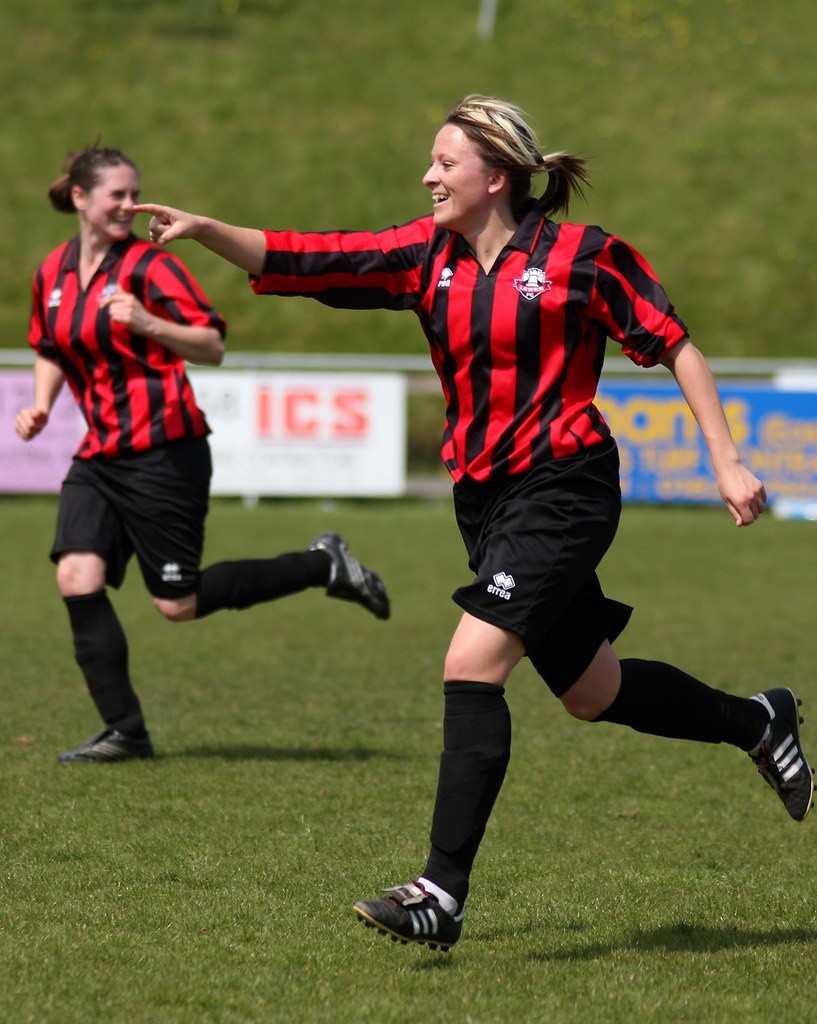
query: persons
[128,93,817,950]
[12,133,392,761]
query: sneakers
[748,687,813,822]
[55,725,156,760]
[352,880,465,953]
[310,532,394,622]
